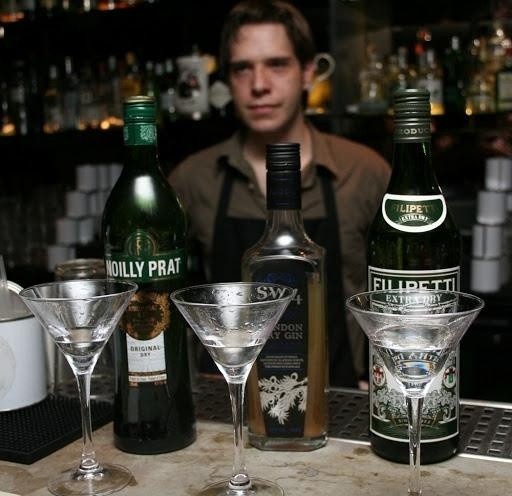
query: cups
[41,160,123,270]
[52,259,117,401]
[468,156,510,292]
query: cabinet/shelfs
[1,0,511,314]
[336,321,512,399]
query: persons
[166,1,394,392]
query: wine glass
[167,279,297,496]
[343,288,485,496]
[20,281,139,493]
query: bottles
[357,13,511,117]
[241,142,332,456]
[361,90,463,465]
[99,92,195,456]
[0,0,215,135]
